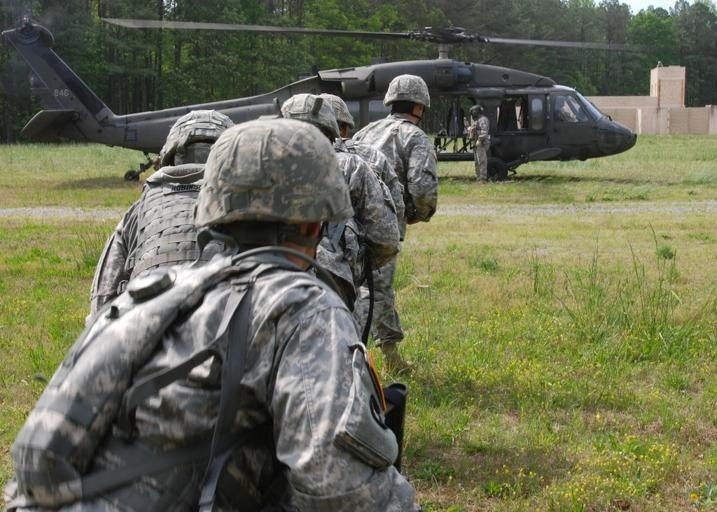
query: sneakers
[475,179,489,186]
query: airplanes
[0,17,668,181]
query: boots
[379,343,414,381]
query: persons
[352,75,439,379]
[461,116,471,152]
[90,111,236,314]
[468,103,492,185]
[320,92,405,342]
[5,116,424,512]
[276,94,400,313]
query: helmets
[194,118,355,230]
[319,91,354,129]
[279,93,341,139]
[383,73,430,108]
[159,110,236,168]
